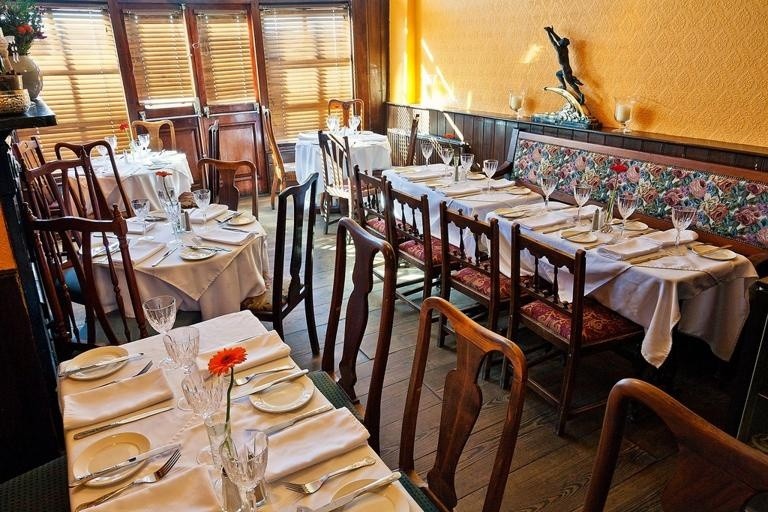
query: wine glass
[670,205,696,255]
[141,294,270,511]
[421,143,434,170]
[440,148,455,176]
[460,153,475,182]
[540,175,558,214]
[130,187,211,245]
[616,193,639,242]
[483,160,498,194]
[613,97,633,133]
[325,116,361,142]
[95,133,152,173]
[573,184,592,226]
[508,89,528,122]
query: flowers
[1,0,48,56]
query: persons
[543,24,584,105]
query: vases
[12,55,44,101]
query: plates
[249,372,316,410]
[467,173,485,180]
[312,140,320,145]
[561,230,598,243]
[73,432,151,487]
[329,479,412,512]
[613,219,649,231]
[92,207,256,269]
[494,207,525,218]
[65,346,130,380]
[691,243,737,262]
[505,187,531,195]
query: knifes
[68,442,182,489]
[73,406,174,440]
[261,406,333,435]
[543,225,578,234]
[315,470,402,512]
[452,192,480,200]
[561,230,596,239]
[230,368,309,400]
[499,209,528,217]
[58,352,143,377]
[547,204,578,212]
[413,178,440,183]
[631,252,672,265]
[628,228,661,239]
[697,244,733,256]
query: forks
[282,458,376,494]
[224,365,295,386]
[73,449,182,512]
[583,236,619,250]
[93,361,153,389]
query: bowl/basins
[0,89,31,114]
